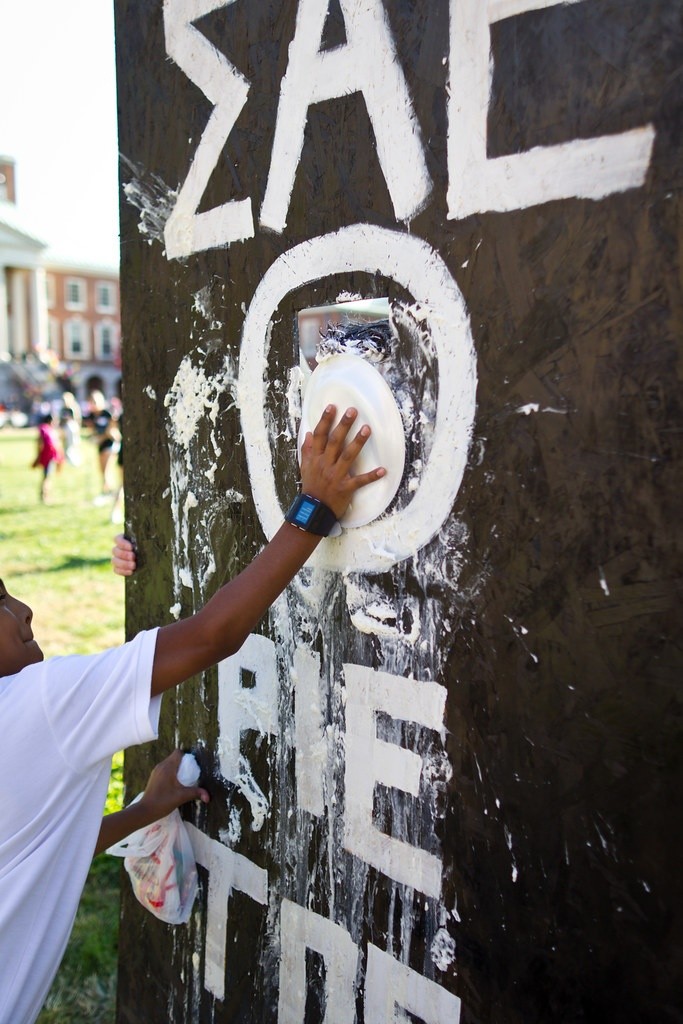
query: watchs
[285,494,336,537]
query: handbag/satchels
[104,753,200,924]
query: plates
[298,354,405,530]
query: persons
[31,390,122,503]
[112,534,136,575]
[1,403,387,1024]
